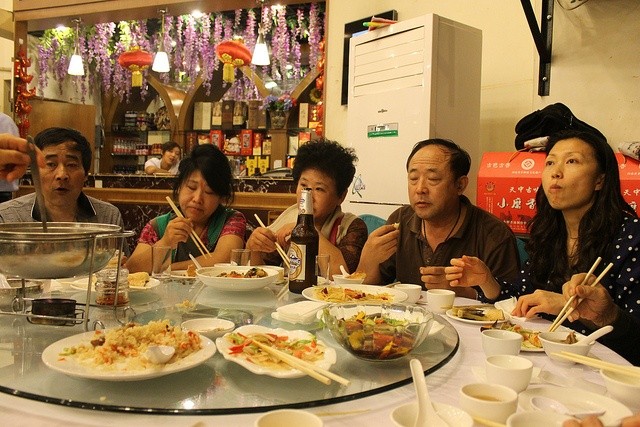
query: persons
[1,127,130,258]
[355,138,521,301]
[241,139,368,286]
[0,134,45,183]
[126,144,247,274]
[144,142,181,175]
[562,273,640,361]
[445,131,640,342]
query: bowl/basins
[269,265,285,278]
[390,400,473,426]
[181,317,235,339]
[506,409,583,427]
[538,331,596,365]
[600,365,640,407]
[332,275,365,284]
[323,301,434,362]
[0,281,46,312]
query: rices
[82,318,203,367]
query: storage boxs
[478,152,546,237]
[614,152,640,218]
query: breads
[127,272,150,287]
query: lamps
[67,17,85,76]
[151,8,172,74]
[250,0,271,66]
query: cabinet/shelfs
[285,128,325,177]
[183,127,271,178]
[108,127,170,174]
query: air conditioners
[342,13,482,219]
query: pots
[1,219,122,280]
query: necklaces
[422,205,462,244]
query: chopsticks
[548,256,614,332]
[254,213,290,268]
[165,195,212,261]
[550,350,640,379]
[252,338,351,387]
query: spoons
[409,358,449,427]
[530,396,606,418]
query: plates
[70,276,160,292]
[520,330,544,353]
[446,307,507,325]
[518,387,632,427]
[196,265,279,291]
[171,270,197,280]
[215,324,337,379]
[301,284,409,306]
[41,328,217,383]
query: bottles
[112,135,163,155]
[111,123,124,134]
[112,164,145,173]
[96,252,129,304]
[289,188,319,294]
[124,109,153,130]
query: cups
[480,330,522,356]
[254,408,323,427]
[152,242,172,279]
[395,284,423,304]
[459,383,518,423]
[427,289,456,316]
[230,249,251,266]
[484,354,533,393]
[316,254,331,285]
